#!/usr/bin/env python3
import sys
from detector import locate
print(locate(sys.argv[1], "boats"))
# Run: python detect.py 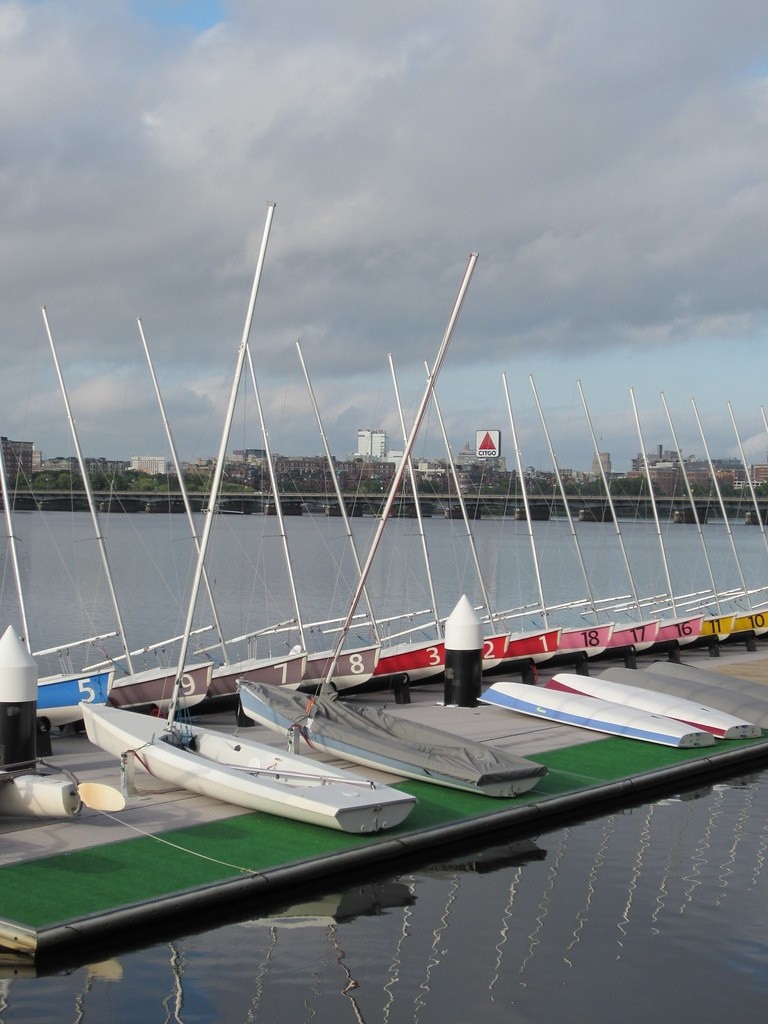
[544,671,763,740]
[232,250,550,800]
[1,304,767,736]
[75,203,420,836]
[475,680,718,749]
